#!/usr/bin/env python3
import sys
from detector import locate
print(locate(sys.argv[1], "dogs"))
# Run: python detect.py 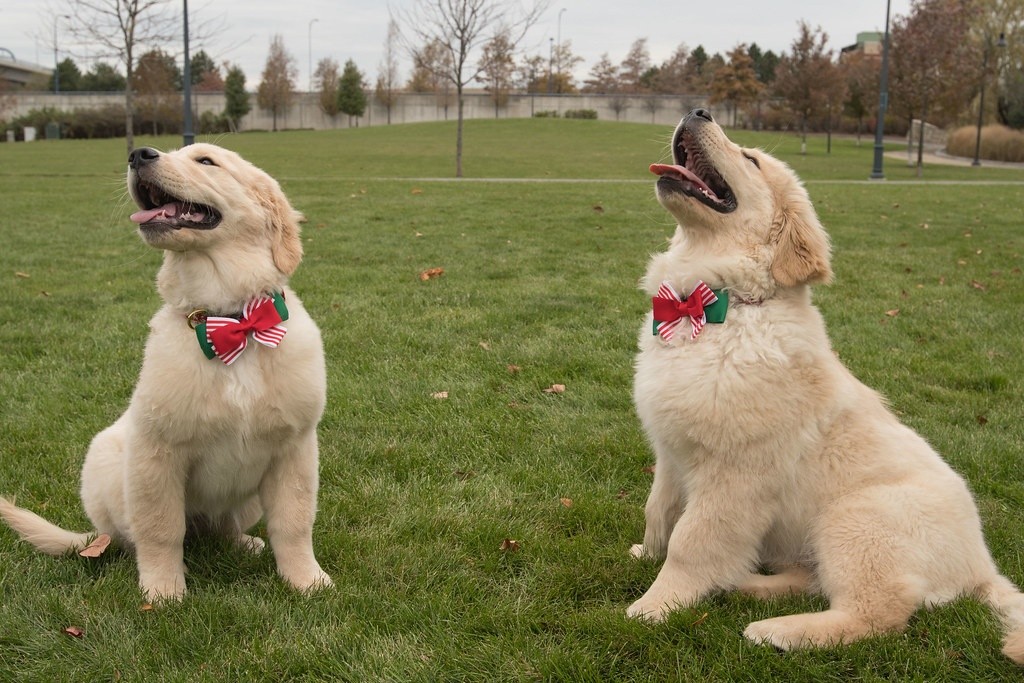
[625,106,1023,666]
[0,141,336,607]
